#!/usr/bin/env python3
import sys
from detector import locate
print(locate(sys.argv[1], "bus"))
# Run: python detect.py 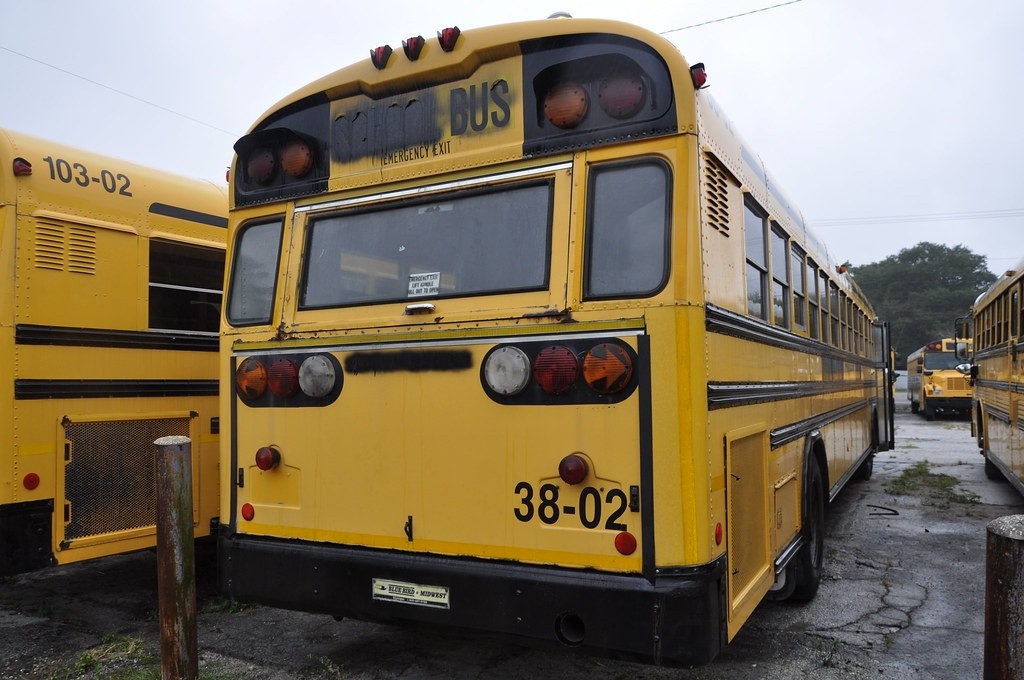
[0,127,452,566]
[948,260,1024,496]
[906,336,977,420]
[209,21,900,659]
